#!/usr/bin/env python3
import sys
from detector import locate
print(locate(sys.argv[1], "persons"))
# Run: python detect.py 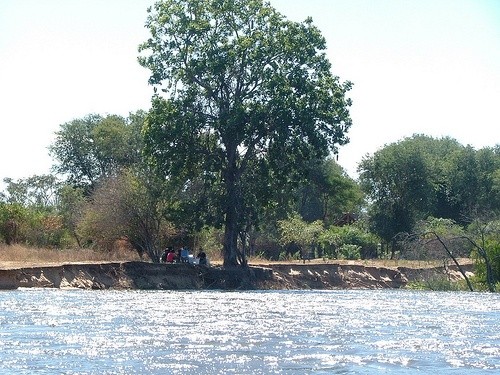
[196,247,207,265]
[181,247,190,263]
[161,249,169,262]
[166,249,176,263]
[176,246,183,261]
